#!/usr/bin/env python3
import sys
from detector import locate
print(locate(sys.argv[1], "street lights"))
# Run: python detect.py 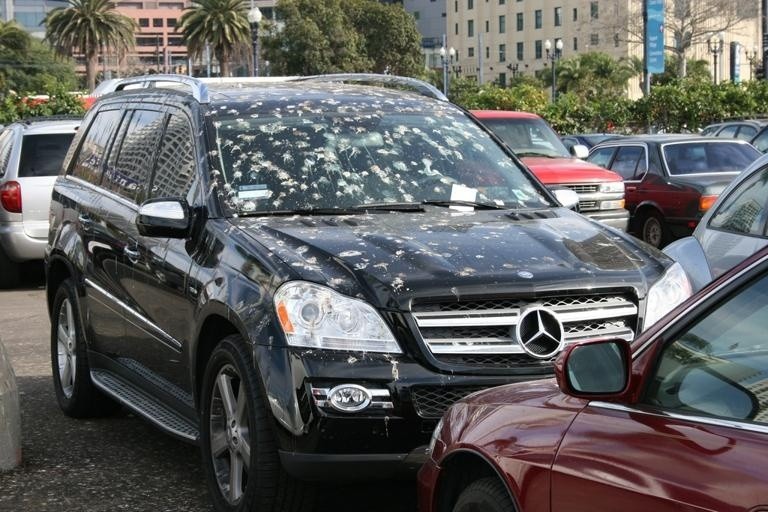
[744,44,759,81]
[439,45,456,99]
[544,38,564,104]
[706,34,724,85]
[246,5,266,77]
